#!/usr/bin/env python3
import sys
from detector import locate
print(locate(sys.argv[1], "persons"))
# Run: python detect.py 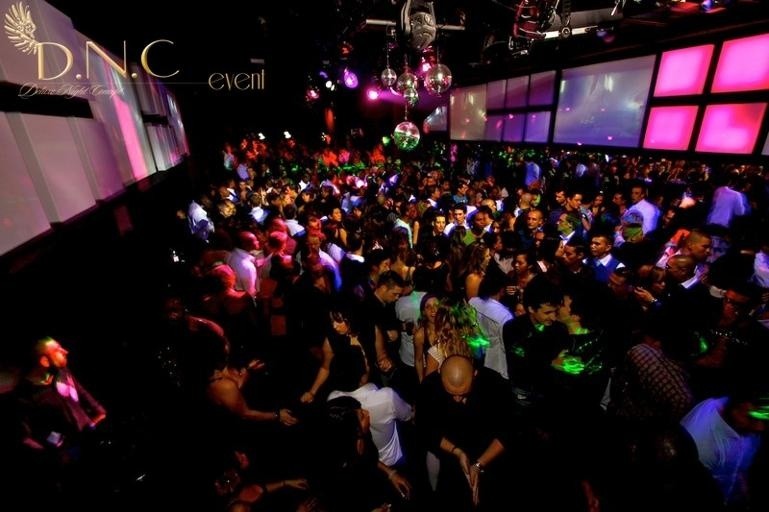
[9,134,768,511]
